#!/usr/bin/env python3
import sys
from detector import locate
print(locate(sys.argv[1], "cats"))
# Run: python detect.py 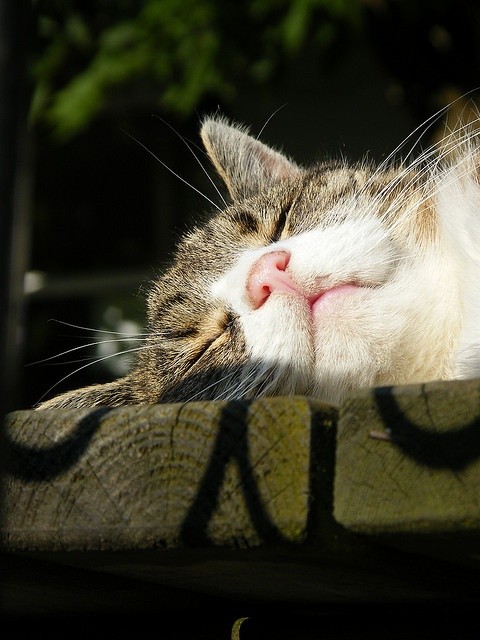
[23,87,480,414]
